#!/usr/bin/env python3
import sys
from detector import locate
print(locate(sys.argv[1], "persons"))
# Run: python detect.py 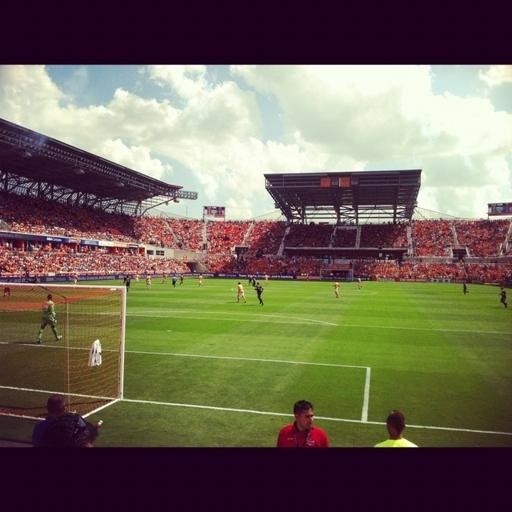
[373,408,422,448]
[1,188,512,297]
[35,294,63,344]
[236,280,248,304]
[498,286,509,308]
[33,394,85,450]
[75,419,100,447]
[254,279,264,303]
[277,399,331,448]
